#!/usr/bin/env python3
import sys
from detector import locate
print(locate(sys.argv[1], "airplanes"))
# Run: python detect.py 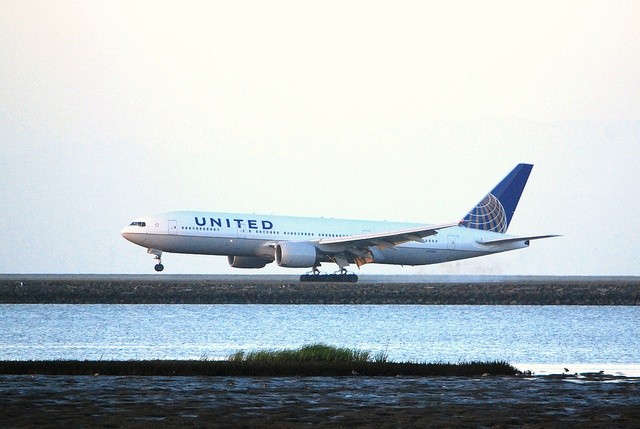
[122,163,563,281]
